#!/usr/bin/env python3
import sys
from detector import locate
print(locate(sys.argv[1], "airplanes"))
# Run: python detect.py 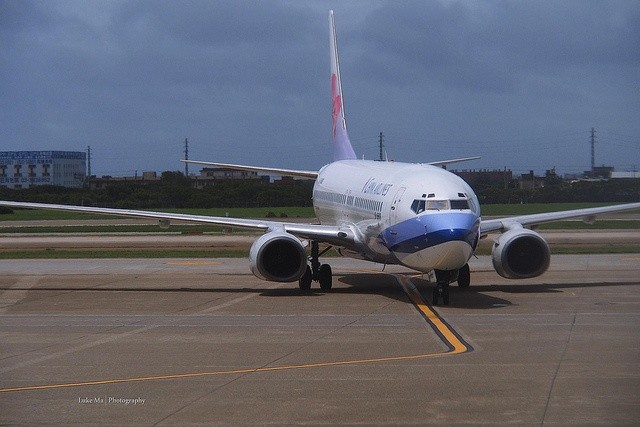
[0,10,627,306]
